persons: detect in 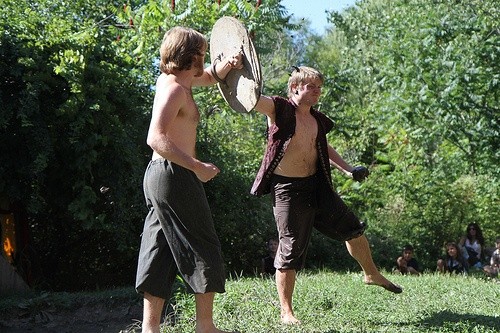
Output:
[136,26,243,333]
[436,223,500,278]
[250,66,403,326]
[389,245,426,275]
